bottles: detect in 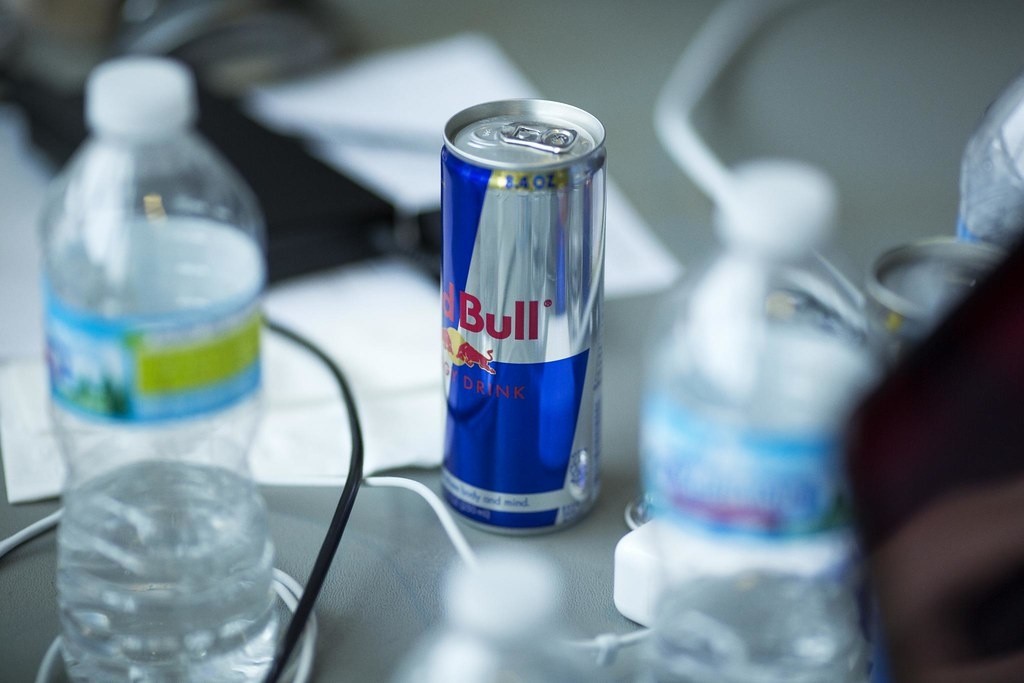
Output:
[37,57,276,683]
[958,79,1024,246]
[640,161,883,683]
